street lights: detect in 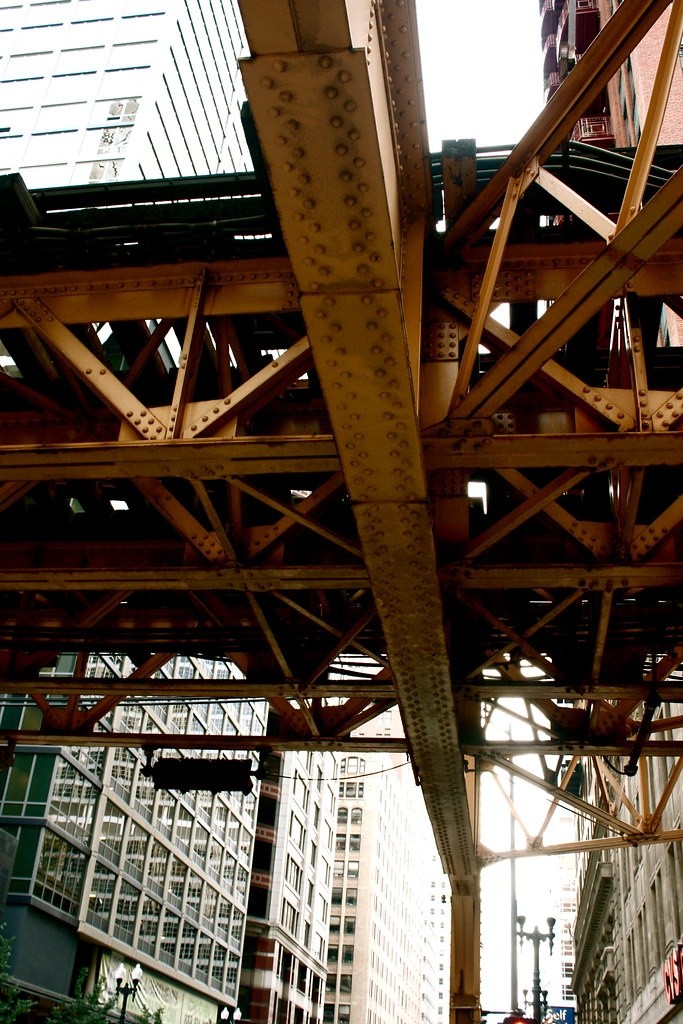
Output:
[114,963,143,1024]
[515,914,555,1023]
